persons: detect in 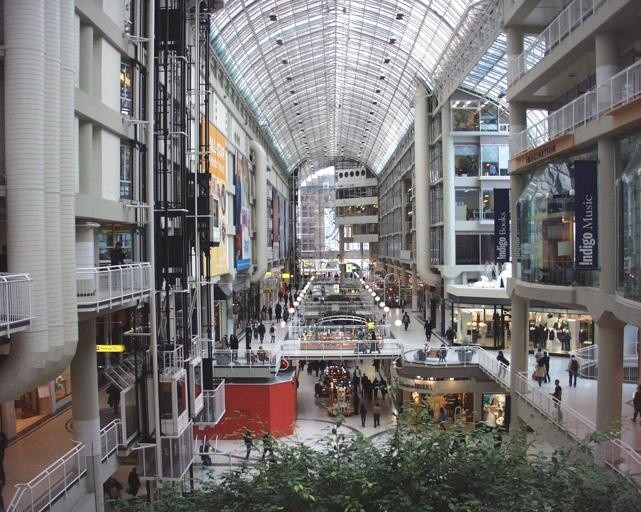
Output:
[297,357,403,429]
[222,275,307,364]
[221,184,227,215]
[445,327,457,347]
[127,467,141,502]
[105,476,122,512]
[400,296,405,313]
[423,316,433,342]
[105,379,122,415]
[217,184,222,199]
[330,210,336,225]
[200,437,211,470]
[484,397,504,427]
[321,287,325,296]
[357,313,386,354]
[549,379,562,420]
[449,427,466,454]
[402,312,410,331]
[424,343,447,363]
[498,352,507,378]
[492,426,503,451]
[261,431,275,463]
[110,240,128,291]
[309,289,312,298]
[333,273,353,279]
[530,346,550,387]
[567,354,580,388]
[245,429,255,459]
[631,387,640,423]
[221,222,228,242]
[439,407,447,430]
